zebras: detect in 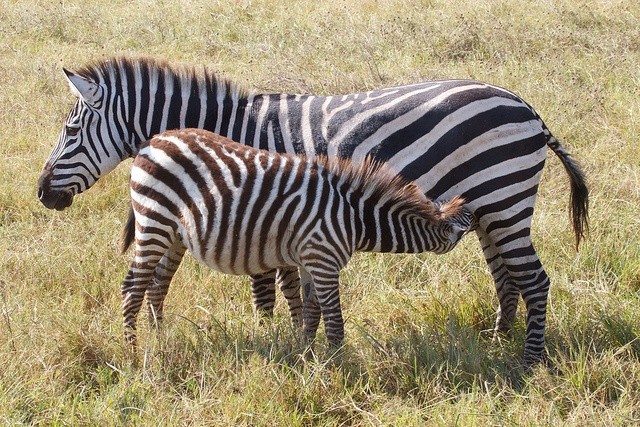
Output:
[118,128,480,391]
[35,54,592,399]
